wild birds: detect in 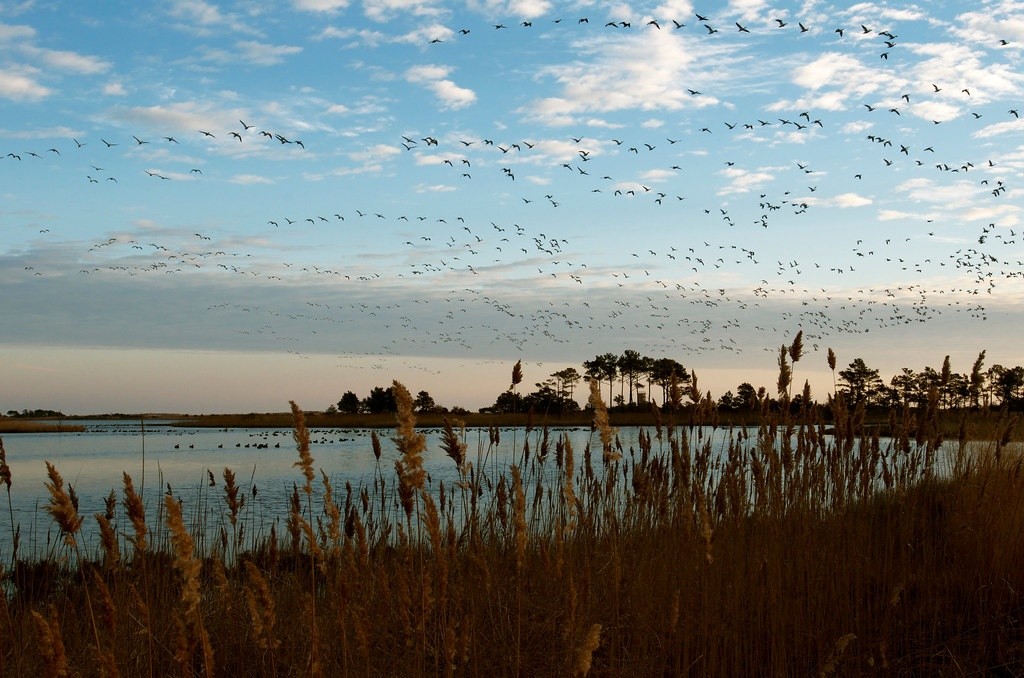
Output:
[0,1,1024,449]
